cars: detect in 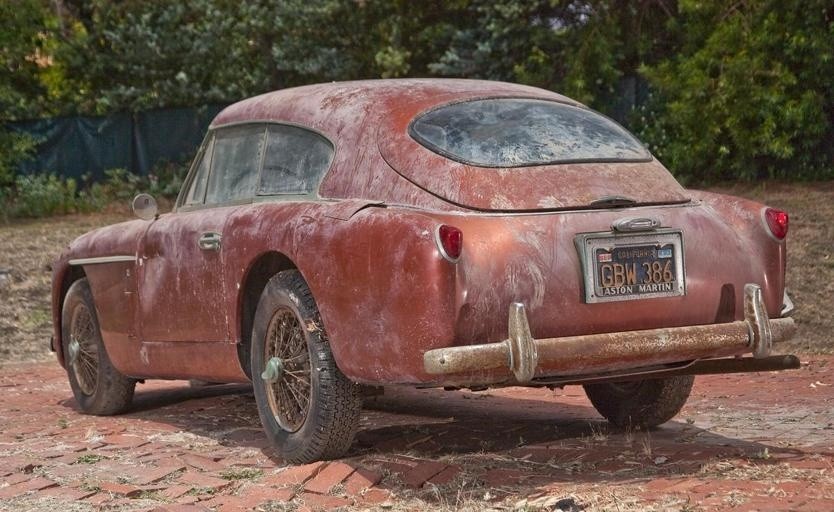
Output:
[48,76,800,464]
